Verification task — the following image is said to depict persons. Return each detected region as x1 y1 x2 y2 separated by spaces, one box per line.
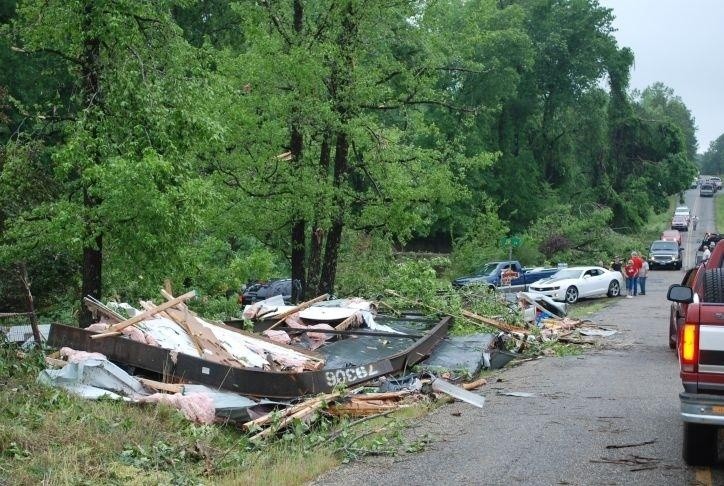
700 232 720 263
692 215 700 231
611 251 650 297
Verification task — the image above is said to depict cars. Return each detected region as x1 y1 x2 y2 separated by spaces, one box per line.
671 206 690 231
691 176 722 197
240 279 314 304
649 239 685 269
660 229 681 244
529 266 623 303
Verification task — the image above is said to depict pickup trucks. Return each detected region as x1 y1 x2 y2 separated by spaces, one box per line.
453 261 558 295
668 239 724 466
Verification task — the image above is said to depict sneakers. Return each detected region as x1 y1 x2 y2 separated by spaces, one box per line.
639 291 645 296
